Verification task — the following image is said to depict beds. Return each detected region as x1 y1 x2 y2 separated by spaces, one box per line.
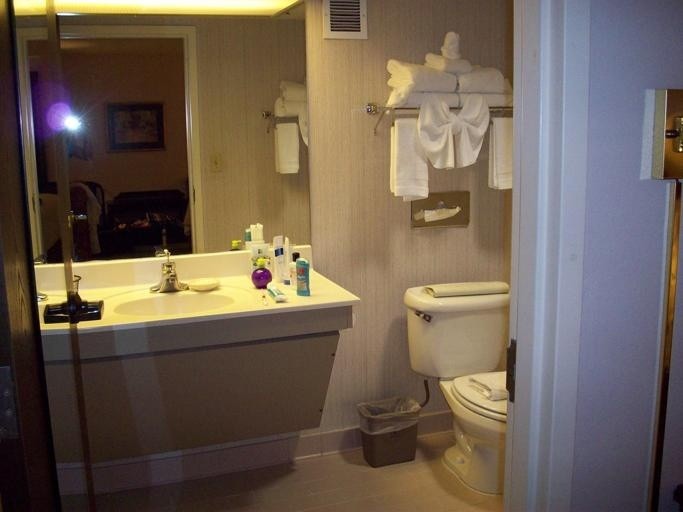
37 179 100 257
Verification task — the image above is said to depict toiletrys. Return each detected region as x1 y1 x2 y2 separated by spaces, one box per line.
228 222 266 249
249 235 311 306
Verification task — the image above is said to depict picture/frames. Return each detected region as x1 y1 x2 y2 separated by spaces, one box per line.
103 100 167 154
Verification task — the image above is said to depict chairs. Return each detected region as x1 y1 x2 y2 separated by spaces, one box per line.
83 181 106 221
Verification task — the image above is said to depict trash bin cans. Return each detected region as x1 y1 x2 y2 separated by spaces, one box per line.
356 395 421 467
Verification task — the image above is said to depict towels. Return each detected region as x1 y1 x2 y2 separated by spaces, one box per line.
466 371 506 401
270 75 310 177
381 25 514 205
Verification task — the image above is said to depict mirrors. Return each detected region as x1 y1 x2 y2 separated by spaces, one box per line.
15 23 205 264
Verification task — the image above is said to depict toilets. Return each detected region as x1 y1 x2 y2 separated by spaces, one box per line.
404 281 510 495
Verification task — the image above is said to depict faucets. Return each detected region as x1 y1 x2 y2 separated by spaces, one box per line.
153 245 170 256
162 272 178 286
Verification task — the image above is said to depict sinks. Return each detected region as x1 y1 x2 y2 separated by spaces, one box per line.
113 291 235 318
